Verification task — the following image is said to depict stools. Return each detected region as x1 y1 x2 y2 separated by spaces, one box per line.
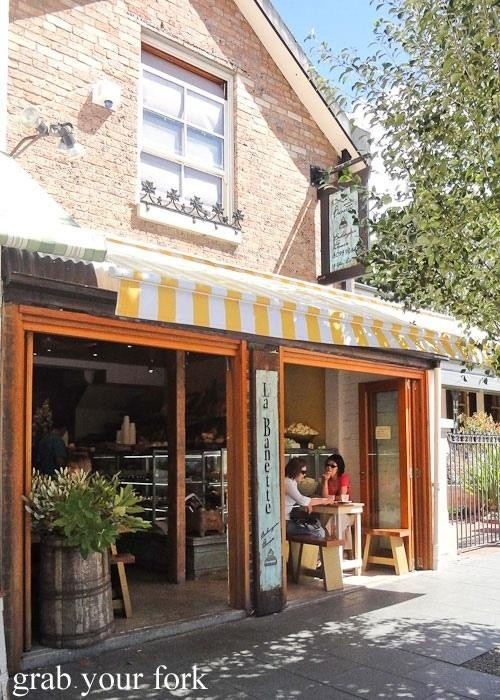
363 526 410 576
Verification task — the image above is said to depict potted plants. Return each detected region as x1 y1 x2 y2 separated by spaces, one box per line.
20 460 152 649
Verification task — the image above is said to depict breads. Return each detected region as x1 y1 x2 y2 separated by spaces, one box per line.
201 429 224 444
298 477 314 484
284 423 319 436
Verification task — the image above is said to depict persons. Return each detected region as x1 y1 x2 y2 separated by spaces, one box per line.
67 454 93 480
320 455 356 539
284 458 328 579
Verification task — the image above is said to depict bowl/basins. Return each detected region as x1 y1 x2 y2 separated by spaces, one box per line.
285 433 319 448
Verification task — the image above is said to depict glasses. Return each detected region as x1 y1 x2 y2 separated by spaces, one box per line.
325 463 338 468
301 470 308 475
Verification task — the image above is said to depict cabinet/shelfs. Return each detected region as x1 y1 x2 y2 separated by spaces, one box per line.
94 451 335 581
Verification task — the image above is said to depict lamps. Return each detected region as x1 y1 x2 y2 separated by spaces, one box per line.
10 106 85 162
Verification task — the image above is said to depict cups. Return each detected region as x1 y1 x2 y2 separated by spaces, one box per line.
328 495 336 501
341 495 349 502
115 415 137 445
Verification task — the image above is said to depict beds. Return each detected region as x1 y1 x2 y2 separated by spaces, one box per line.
300 502 365 578
